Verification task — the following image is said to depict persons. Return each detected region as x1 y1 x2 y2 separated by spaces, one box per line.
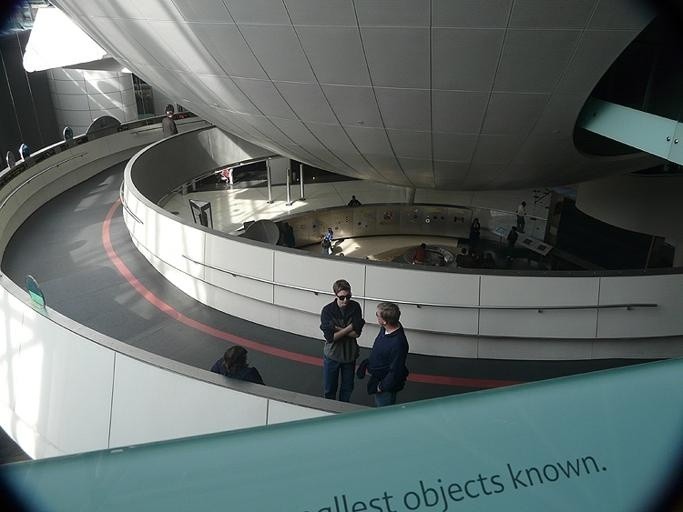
455 247 473 267
160 110 178 138
325 231 333 255
282 221 296 248
211 345 266 385
478 253 496 268
323 227 332 242
410 242 427 265
318 279 366 403
355 301 409 408
346 195 361 207
469 217 481 239
504 225 518 247
517 201 527 233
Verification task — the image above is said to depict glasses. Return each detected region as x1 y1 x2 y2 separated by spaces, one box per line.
336 294 351 300
